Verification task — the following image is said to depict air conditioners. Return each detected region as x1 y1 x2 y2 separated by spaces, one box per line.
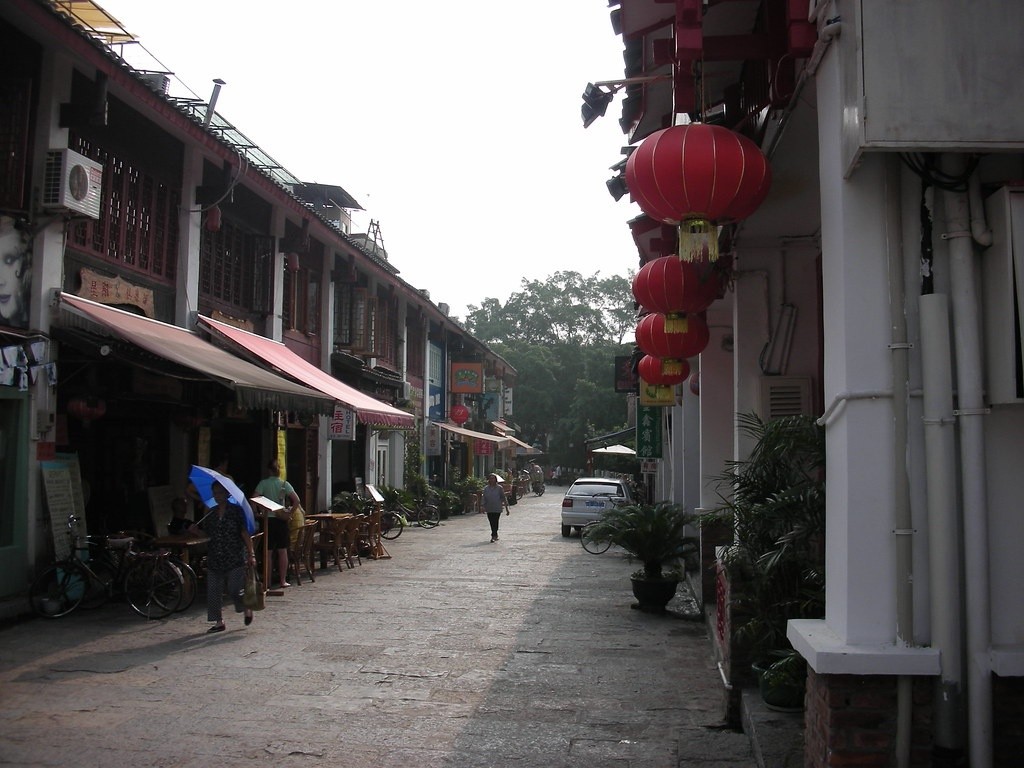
40 147 102 220
295 409 320 427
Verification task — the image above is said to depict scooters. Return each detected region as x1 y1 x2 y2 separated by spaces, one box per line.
515 471 546 498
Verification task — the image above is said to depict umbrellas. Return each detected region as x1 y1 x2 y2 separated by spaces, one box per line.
183 464 257 538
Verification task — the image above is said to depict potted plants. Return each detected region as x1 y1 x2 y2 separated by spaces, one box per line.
583 496 702 606
698 408 830 711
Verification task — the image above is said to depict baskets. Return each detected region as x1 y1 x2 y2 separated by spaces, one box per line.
48 533 74 567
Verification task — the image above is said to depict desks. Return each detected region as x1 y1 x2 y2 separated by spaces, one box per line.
306 512 351 568
151 535 210 602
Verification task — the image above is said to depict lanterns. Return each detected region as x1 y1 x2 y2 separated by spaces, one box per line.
637 355 699 399
631 254 720 332
207 205 221 232
288 251 298 272
450 405 468 424
625 122 772 262
635 314 710 376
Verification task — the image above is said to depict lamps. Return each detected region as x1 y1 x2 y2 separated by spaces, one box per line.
636 305 648 318
581 73 673 128
607 175 626 202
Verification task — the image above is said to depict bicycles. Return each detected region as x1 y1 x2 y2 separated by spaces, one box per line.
29 512 183 624
376 488 441 531
346 490 404 540
62 532 199 614
580 497 639 554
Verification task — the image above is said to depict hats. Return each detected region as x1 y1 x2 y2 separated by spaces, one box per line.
254 455 279 474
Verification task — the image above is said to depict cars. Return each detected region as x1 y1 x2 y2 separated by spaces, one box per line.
561 477 636 537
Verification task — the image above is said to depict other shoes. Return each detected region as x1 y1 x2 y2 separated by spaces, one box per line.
209 626 225 633
244 609 253 625
281 582 291 587
491 537 499 542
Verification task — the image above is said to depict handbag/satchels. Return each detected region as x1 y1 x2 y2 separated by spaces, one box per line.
235 570 264 613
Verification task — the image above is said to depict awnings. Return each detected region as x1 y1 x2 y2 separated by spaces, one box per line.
432 421 533 450
584 427 636 452
197 314 416 427
60 292 335 419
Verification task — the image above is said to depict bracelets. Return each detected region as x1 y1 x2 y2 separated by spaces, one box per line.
247 552 256 557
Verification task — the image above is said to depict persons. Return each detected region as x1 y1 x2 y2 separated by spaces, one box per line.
505 463 561 493
480 475 510 542
188 480 256 633
185 452 233 515
0 214 33 330
605 472 637 493
252 460 306 588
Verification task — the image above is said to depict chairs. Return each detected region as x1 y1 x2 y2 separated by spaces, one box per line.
250 510 386 586
